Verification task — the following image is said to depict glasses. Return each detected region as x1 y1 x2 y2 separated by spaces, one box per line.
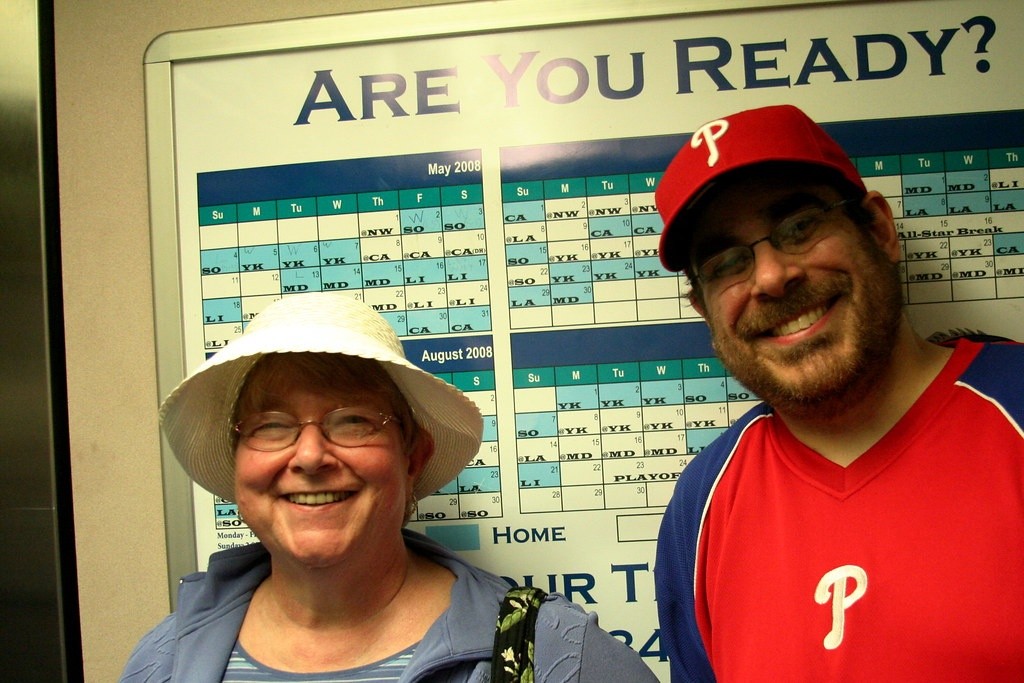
692 193 856 299
230 406 405 453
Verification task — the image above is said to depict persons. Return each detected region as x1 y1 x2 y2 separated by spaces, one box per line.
654 105 1024 683
118 292 660 683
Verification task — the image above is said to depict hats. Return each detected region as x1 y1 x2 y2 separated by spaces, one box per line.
153 289 485 504
652 104 870 272
